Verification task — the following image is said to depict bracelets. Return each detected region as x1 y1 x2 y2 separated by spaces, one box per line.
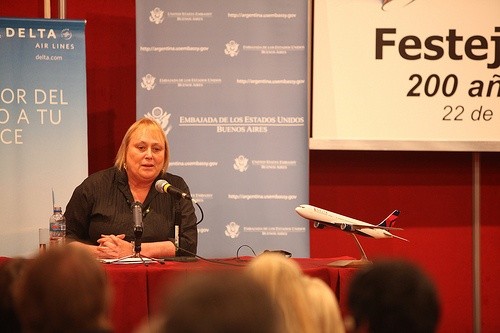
131 242 136 255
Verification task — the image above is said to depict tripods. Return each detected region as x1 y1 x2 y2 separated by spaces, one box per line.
107 221 164 267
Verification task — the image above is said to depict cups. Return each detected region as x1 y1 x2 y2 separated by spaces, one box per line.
38 228 49 252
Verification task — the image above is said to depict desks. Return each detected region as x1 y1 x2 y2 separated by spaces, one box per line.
0 256 360 333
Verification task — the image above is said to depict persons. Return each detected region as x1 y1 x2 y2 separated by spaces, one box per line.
249 254 346 333
346 259 440 333
0 247 104 333
60 118 198 259
167 267 286 333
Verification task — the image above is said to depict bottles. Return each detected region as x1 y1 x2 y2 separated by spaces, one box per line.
49 206 66 250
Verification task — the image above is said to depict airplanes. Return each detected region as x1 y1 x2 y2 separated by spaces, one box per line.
293 203 409 260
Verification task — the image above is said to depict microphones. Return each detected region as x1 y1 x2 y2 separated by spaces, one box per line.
130 201 145 236
155 179 192 200
